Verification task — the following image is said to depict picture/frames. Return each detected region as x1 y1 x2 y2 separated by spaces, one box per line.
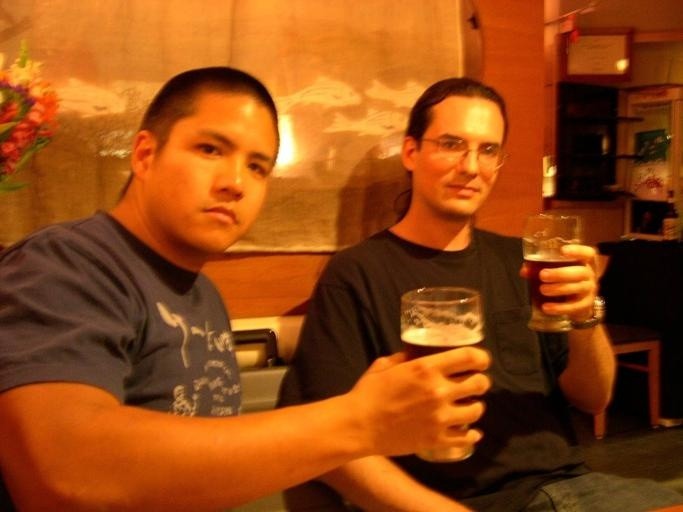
558 26 634 85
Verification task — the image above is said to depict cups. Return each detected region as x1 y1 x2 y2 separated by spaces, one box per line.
520 211 581 333
398 284 487 466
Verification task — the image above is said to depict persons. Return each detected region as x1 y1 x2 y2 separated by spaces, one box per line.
0 65 494 512
274 73 682 512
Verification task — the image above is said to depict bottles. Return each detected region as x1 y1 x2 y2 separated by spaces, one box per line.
661 189 681 241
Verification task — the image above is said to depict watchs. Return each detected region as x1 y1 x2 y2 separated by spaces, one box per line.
569 295 607 333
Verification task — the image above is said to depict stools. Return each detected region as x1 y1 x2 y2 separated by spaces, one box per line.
592 323 663 441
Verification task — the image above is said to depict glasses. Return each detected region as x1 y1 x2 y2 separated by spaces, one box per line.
416 135 508 172
